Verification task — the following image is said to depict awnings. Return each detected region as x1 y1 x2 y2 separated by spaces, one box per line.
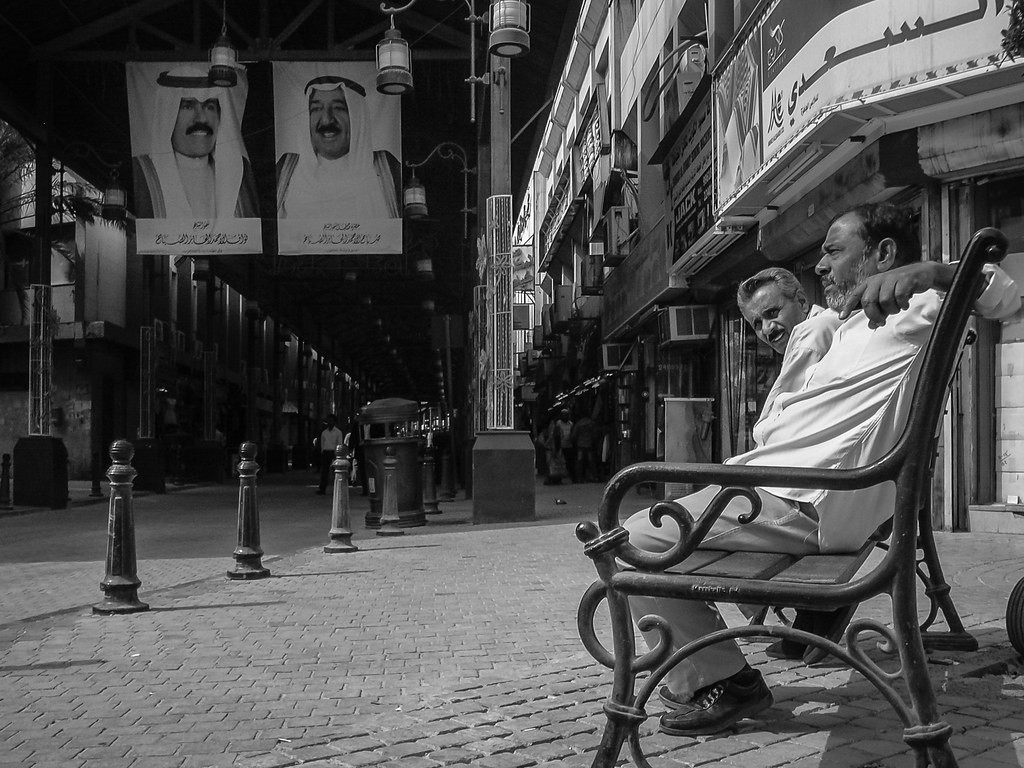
547 372 615 412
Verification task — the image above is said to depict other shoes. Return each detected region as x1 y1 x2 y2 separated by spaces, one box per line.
765 602 860 665
659 662 773 736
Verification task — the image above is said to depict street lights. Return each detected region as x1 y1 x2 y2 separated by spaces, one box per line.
374 0 535 525
405 141 489 498
12 140 127 508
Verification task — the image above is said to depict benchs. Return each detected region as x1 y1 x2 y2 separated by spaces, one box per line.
575 222 1009 768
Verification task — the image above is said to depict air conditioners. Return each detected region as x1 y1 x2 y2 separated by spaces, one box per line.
602 342 638 370
658 303 717 345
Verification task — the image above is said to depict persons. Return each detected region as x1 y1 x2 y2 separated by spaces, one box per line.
131 66 256 219
276 74 402 219
738 269 858 665
536 408 598 483
315 415 343 494
616 203 1022 735
344 413 371 496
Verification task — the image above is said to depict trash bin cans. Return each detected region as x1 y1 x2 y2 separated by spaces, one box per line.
421 456 443 515
359 397 427 530
437 455 458 503
